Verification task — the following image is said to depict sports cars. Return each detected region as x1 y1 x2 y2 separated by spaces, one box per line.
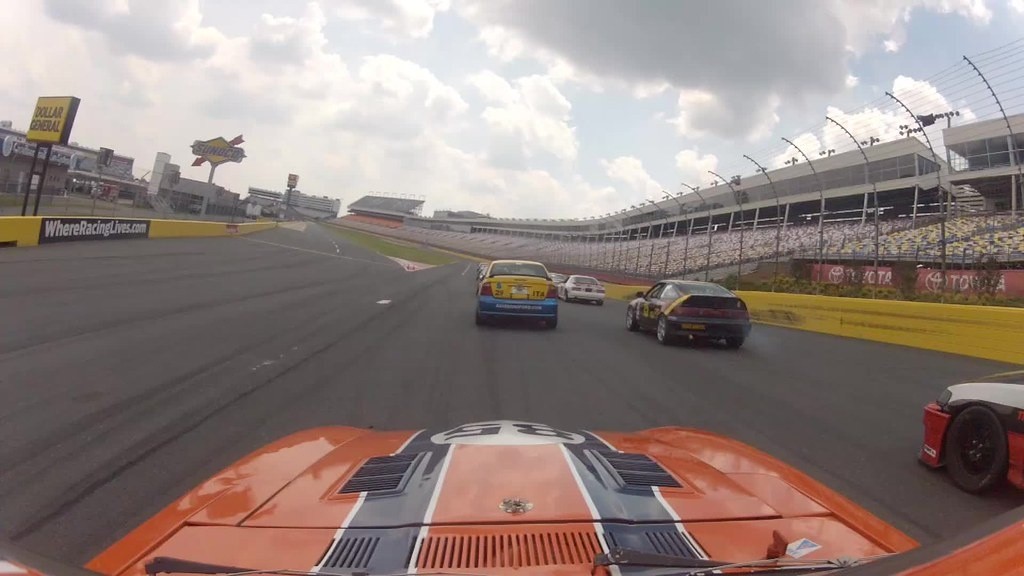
917 365 1024 498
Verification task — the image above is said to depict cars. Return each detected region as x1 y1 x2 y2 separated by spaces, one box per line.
626 280 753 346
474 262 490 292
549 272 567 291
557 274 607 306
474 260 560 330
